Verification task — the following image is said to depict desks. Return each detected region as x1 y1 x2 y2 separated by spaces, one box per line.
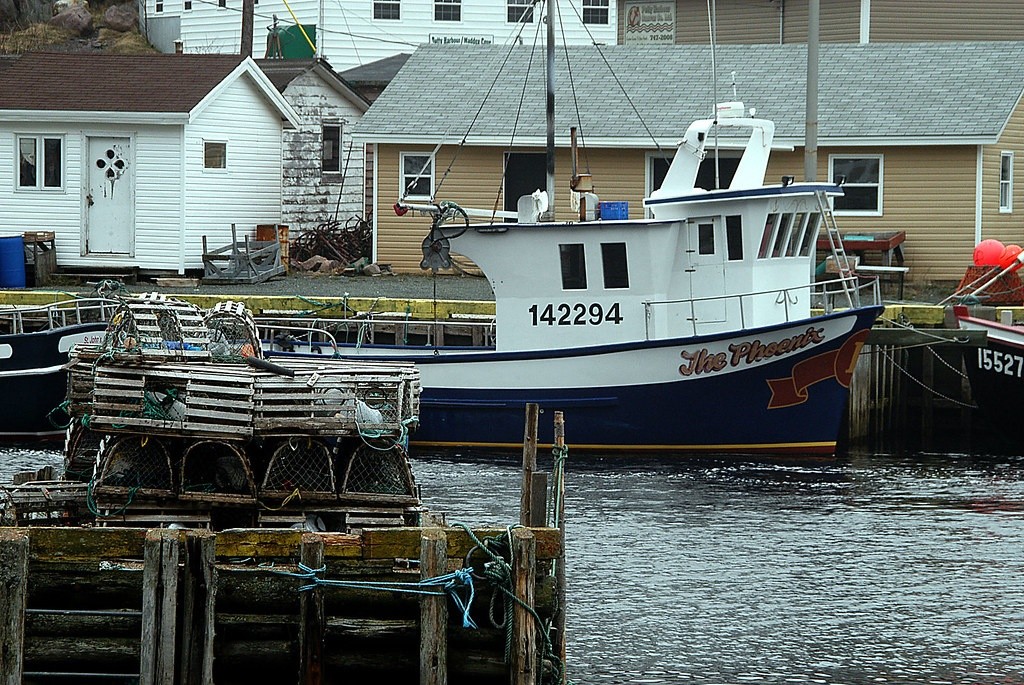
814 230 909 300
202 223 286 284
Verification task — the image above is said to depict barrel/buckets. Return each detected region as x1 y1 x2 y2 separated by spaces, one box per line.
0 236 26 288
257 225 289 277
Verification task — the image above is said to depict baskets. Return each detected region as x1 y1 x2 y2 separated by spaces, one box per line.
600 201 628 220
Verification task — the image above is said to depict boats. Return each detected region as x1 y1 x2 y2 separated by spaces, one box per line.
0 295 132 445
942 253 1024 460
129 1 888 469
